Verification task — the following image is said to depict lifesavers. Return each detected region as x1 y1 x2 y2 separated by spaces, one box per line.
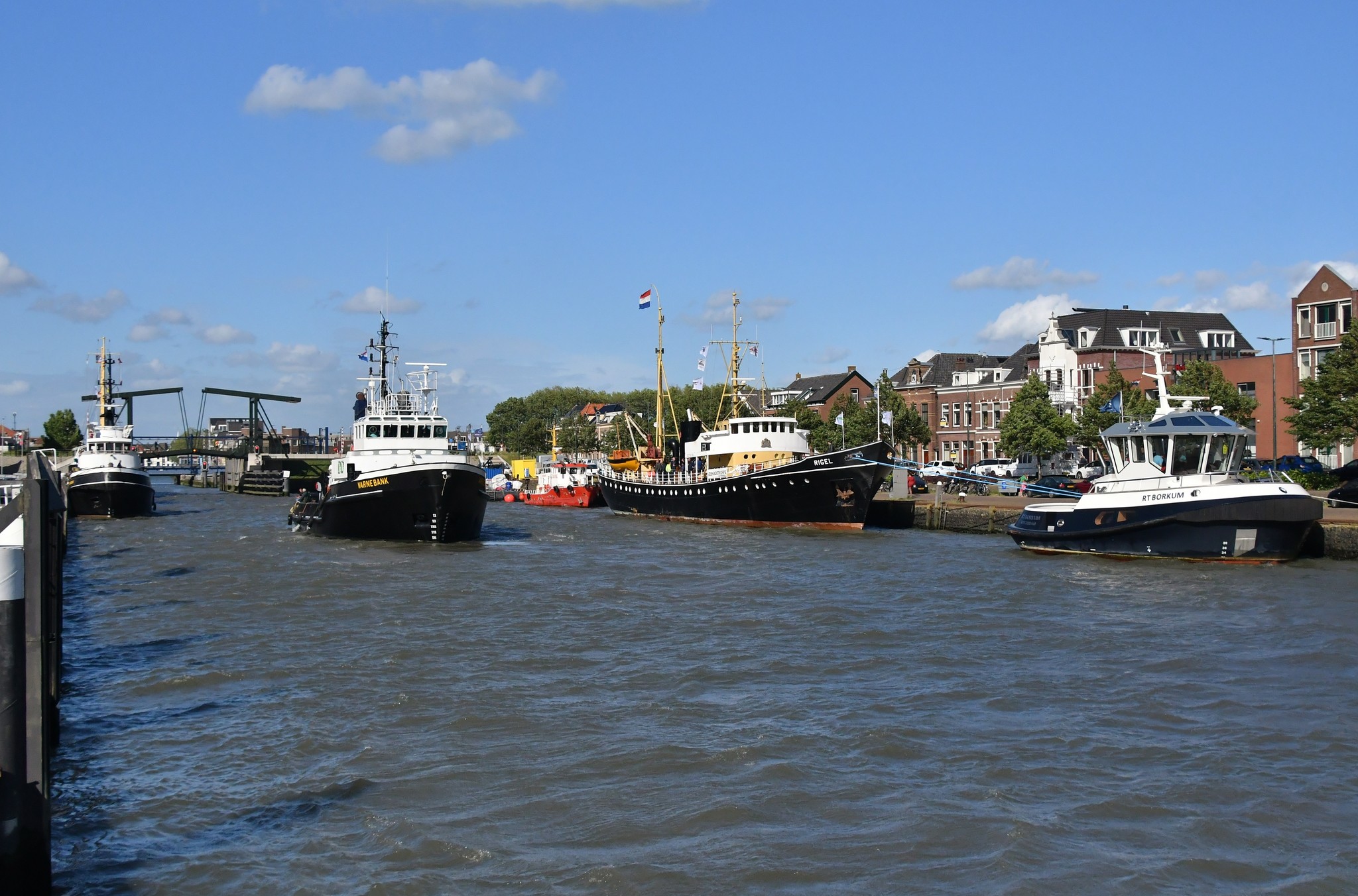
554 486 558 493
296 512 302 523
567 485 573 492
308 516 313 527
584 484 590 489
288 515 293 525
594 484 598 489
527 493 530 499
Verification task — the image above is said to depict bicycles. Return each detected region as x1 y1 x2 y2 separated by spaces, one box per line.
974 478 990 496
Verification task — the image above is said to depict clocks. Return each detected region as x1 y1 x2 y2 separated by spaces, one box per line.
1321 282 1328 292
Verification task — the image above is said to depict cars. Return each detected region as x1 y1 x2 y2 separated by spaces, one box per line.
970 458 1013 476
895 459 920 471
1073 474 1102 500
918 460 958 478
954 462 966 471
1074 459 1116 479
1229 454 1334 475
890 474 929 494
1024 476 1077 498
1328 458 1358 482
1327 478 1358 508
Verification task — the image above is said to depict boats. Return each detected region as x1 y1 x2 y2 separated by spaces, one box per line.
286 252 602 544
61 336 157 520
594 281 897 531
1005 320 1326 563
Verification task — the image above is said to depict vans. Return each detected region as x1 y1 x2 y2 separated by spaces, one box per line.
1005 445 1080 479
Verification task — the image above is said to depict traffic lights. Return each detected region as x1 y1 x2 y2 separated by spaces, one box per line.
254 445 260 455
202 461 208 470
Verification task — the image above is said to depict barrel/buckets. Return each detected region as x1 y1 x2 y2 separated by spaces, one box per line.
506 482 511 489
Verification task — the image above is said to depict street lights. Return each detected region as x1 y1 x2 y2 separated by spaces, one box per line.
1257 337 1291 470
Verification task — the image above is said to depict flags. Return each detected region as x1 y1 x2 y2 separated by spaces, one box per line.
835 412 844 426
639 289 652 309
1131 378 1141 387
749 345 759 358
873 388 879 399
358 350 368 362
692 376 704 391
880 411 893 428
474 428 483 439
697 358 706 372
699 345 709 358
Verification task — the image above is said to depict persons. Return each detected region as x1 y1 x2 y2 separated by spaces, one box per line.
889 477 893 492
165 442 168 452
907 473 915 497
154 441 158 452
941 473 959 494
117 460 122 468
295 488 310 504
1020 480 1028 497
1153 453 1166 468
215 439 219 450
828 440 835 453
654 457 707 485
1018 473 1028 496
1099 391 1123 415
